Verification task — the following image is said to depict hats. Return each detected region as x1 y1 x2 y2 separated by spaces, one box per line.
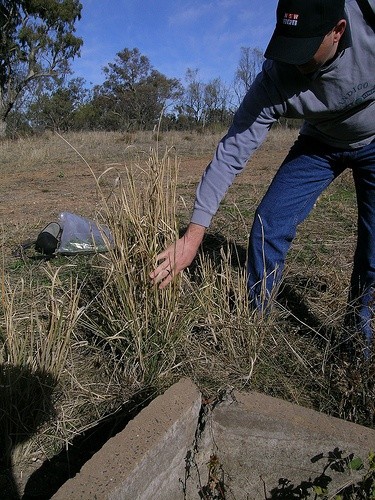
264 1 346 64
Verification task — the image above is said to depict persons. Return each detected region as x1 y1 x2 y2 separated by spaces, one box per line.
148 0 375 364
